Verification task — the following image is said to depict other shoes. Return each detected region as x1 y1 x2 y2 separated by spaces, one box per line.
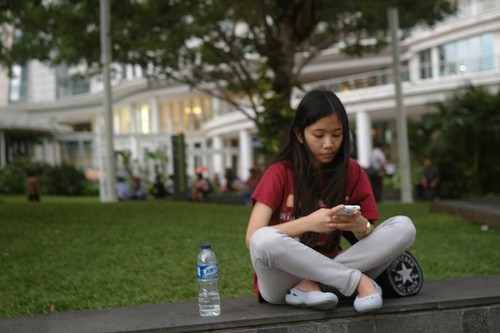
284 287 338 309
354 277 383 313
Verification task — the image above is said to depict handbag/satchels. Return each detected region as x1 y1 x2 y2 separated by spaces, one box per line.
376 250 424 298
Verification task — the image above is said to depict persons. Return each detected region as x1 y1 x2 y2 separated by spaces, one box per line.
115 142 440 203
246 90 417 311
27 171 40 200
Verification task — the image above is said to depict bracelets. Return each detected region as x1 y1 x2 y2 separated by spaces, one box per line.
357 220 371 236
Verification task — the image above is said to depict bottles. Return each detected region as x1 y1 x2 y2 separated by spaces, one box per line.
196 244 221 317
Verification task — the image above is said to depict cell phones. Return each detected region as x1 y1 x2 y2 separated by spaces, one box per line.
336 206 360 216
